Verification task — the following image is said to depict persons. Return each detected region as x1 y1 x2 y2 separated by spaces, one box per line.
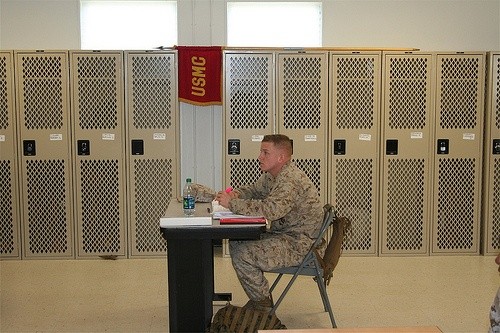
216 133 324 313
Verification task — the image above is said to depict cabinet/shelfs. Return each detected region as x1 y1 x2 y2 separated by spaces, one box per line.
223 45 500 260
0 48 179 257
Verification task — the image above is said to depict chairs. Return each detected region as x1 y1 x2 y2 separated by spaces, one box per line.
269 203 337 328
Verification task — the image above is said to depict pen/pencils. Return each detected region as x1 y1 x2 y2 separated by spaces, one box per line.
207 207 210 213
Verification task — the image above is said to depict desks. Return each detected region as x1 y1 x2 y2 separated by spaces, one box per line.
160 199 270 333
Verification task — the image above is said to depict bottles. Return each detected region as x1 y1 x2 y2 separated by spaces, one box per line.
182 178 196 216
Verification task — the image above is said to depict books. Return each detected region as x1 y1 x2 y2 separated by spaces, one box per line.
212 201 263 219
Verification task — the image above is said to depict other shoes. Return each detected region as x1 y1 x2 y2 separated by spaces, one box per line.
241 299 275 316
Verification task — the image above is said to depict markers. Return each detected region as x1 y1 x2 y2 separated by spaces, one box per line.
215 187 233 201
